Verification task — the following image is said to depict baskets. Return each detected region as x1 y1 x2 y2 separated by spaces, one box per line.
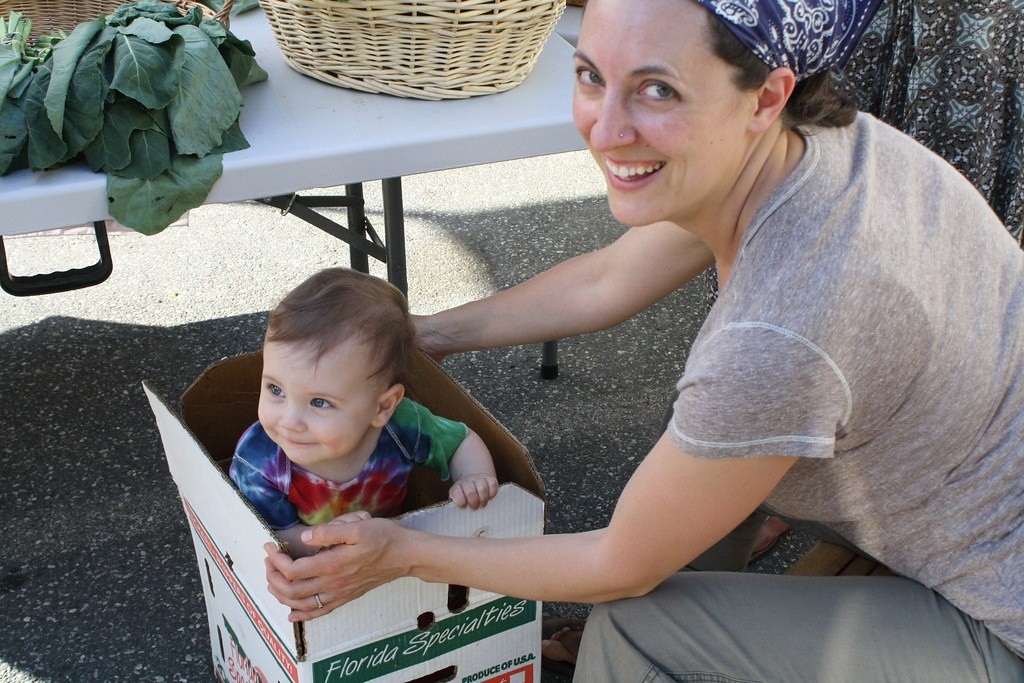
0 0 233 49
258 0 566 100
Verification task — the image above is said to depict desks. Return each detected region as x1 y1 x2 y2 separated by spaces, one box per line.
0 6 590 315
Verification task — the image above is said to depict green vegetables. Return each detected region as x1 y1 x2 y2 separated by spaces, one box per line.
0 0 268 238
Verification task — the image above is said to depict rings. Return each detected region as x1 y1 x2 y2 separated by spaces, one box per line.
314 594 323 608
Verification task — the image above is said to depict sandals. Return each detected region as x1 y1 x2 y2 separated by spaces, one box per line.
542 618 586 675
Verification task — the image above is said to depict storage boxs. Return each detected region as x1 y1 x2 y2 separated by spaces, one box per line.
141 348 548 683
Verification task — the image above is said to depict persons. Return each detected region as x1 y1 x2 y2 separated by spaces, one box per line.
263 0 1024 682
228 267 499 558
750 0 1024 574
